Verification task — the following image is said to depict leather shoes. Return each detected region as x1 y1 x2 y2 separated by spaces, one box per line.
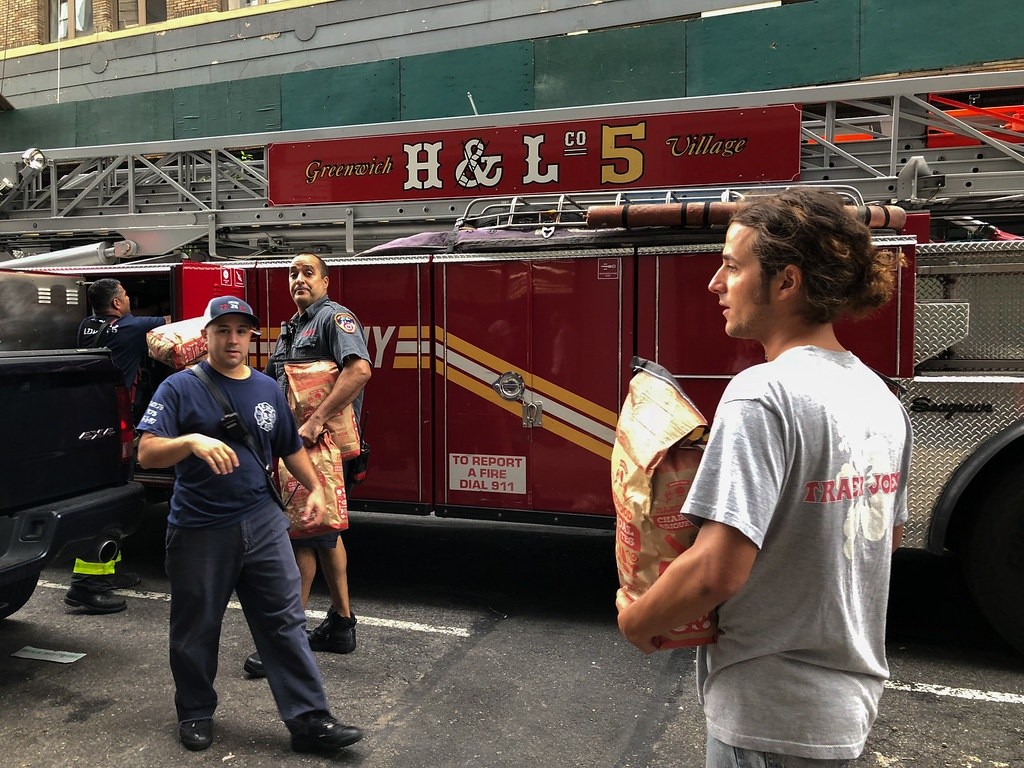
179 719 215 752
286 709 365 752
244 651 266 677
306 605 357 653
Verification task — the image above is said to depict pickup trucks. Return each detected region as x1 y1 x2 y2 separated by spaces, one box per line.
0 346 146 623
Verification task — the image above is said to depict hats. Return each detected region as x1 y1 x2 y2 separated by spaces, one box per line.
203 295 261 329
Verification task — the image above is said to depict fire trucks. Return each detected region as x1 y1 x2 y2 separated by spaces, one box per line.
0 69 1022 668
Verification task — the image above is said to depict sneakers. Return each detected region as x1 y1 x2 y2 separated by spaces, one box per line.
64 573 127 612
101 563 141 589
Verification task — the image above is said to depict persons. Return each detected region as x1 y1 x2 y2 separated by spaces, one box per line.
135 294 364 756
241 249 375 676
615 186 916 768
63 279 177 614
